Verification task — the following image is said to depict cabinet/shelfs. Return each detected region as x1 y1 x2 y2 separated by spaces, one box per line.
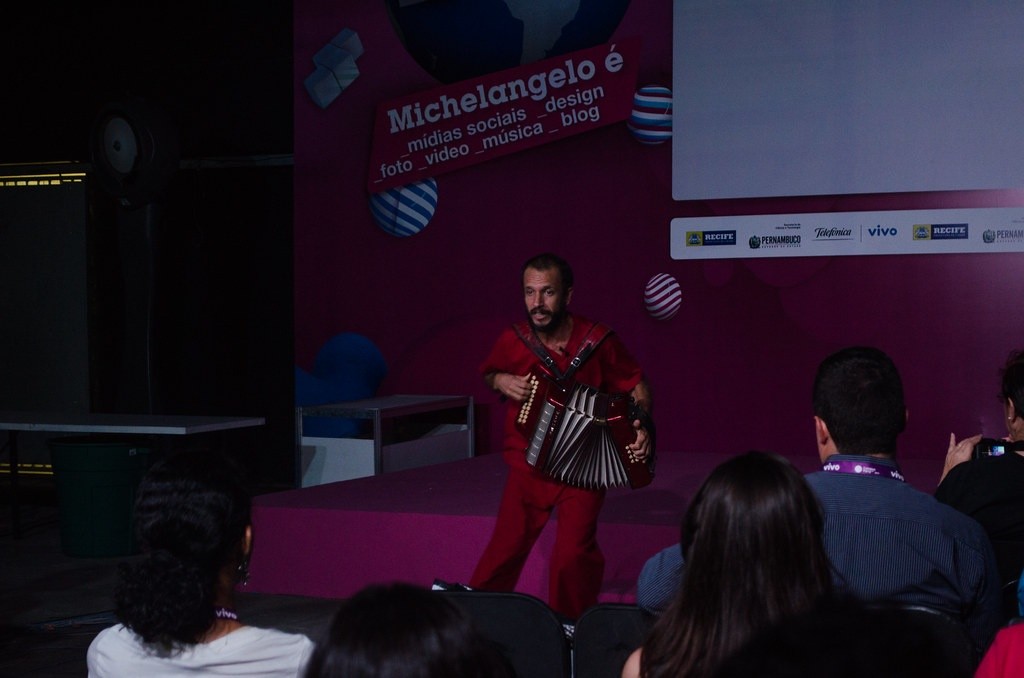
293 391 474 490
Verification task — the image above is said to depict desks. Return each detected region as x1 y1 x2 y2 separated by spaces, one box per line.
0 408 265 541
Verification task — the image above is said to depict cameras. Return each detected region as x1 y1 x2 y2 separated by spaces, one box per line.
972 438 1013 461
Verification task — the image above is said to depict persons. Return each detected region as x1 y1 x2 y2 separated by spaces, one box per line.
622 449 830 678
636 347 1024 678
86 450 317 678
478 253 654 642
934 351 1024 619
301 583 516 678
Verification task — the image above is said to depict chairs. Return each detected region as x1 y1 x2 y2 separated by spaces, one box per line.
573 604 653 678
433 588 571 678
863 604 977 678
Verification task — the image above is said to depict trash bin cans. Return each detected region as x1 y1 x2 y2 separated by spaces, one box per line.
45 437 140 556
